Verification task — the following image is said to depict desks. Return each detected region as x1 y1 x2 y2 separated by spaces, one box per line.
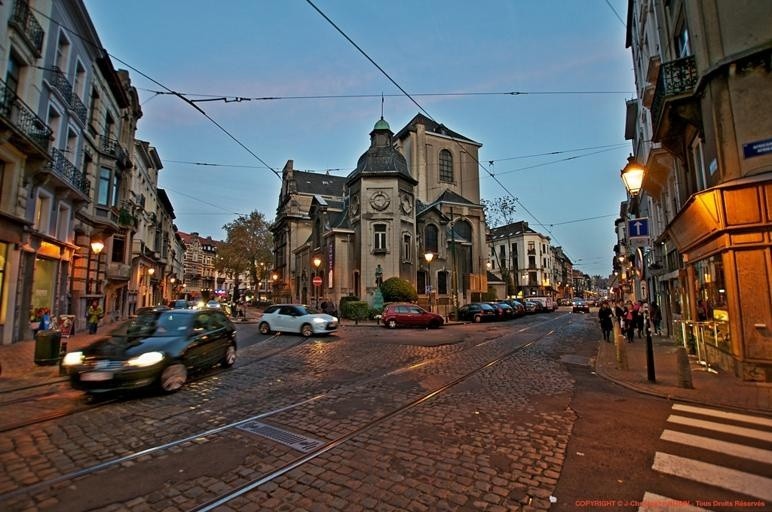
673 320 718 375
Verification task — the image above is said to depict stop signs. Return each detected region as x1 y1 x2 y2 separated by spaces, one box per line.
312 276 323 286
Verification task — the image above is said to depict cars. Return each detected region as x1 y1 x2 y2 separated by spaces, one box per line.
257 303 338 336
59 299 237 395
573 301 589 312
383 303 444 328
446 296 558 322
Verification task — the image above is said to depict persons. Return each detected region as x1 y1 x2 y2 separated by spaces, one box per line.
698 299 713 320
87 300 103 333
598 299 662 344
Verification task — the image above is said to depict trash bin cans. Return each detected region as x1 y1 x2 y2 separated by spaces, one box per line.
33 329 61 366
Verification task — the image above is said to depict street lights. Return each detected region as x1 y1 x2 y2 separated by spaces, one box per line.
313 259 321 308
620 153 655 381
424 250 434 311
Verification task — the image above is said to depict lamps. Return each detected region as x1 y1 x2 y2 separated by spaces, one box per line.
91 240 104 254
